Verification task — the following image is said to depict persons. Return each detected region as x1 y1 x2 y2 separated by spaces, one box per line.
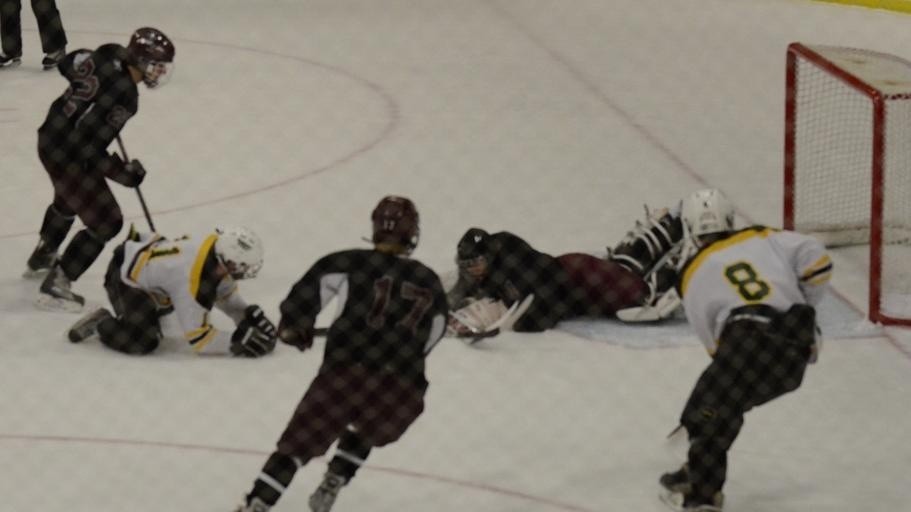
234 192 449 512
657 187 835 512
0 0 68 69
26 26 176 307
435 197 700 344
68 224 277 356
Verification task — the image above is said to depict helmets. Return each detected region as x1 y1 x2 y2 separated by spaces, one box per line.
456 227 499 271
125 27 176 65
679 187 737 237
371 194 420 246
214 221 266 282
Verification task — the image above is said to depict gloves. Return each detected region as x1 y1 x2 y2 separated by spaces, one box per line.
276 301 315 353
105 152 148 187
230 305 277 359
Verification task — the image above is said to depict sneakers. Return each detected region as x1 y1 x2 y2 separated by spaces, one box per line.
27 237 71 271
42 46 65 67
0 47 23 64
309 475 341 510
660 462 724 510
68 308 112 343
39 262 84 307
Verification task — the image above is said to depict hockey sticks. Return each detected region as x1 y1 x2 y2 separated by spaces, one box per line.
313 326 498 340
